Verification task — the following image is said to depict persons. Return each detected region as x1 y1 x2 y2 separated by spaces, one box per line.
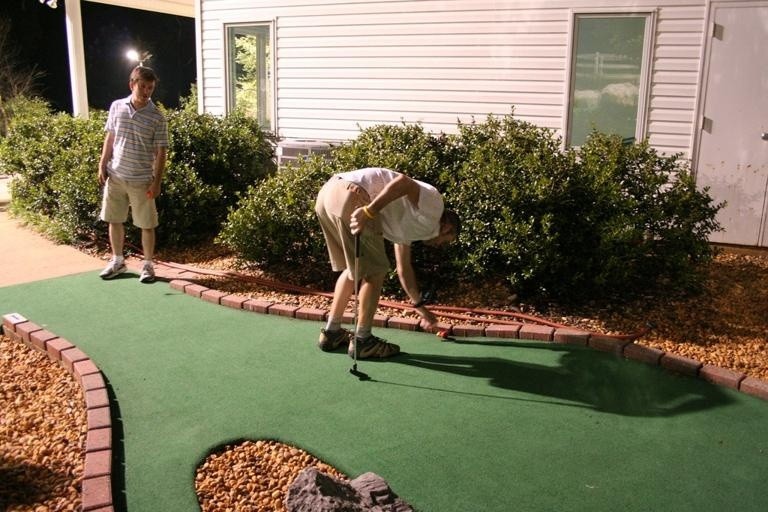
99 65 169 283
315 167 462 360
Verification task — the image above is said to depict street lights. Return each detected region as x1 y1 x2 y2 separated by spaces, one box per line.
126 50 142 67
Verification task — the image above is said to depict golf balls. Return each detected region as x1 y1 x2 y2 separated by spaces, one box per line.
441 331 448 338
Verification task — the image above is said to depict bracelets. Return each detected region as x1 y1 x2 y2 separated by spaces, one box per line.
408 297 424 308
363 205 376 220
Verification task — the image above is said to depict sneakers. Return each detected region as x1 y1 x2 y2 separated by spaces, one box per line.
318 326 355 352
347 334 400 360
99 262 126 279
139 265 155 283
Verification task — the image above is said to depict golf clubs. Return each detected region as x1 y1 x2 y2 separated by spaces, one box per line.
349 232 368 380
